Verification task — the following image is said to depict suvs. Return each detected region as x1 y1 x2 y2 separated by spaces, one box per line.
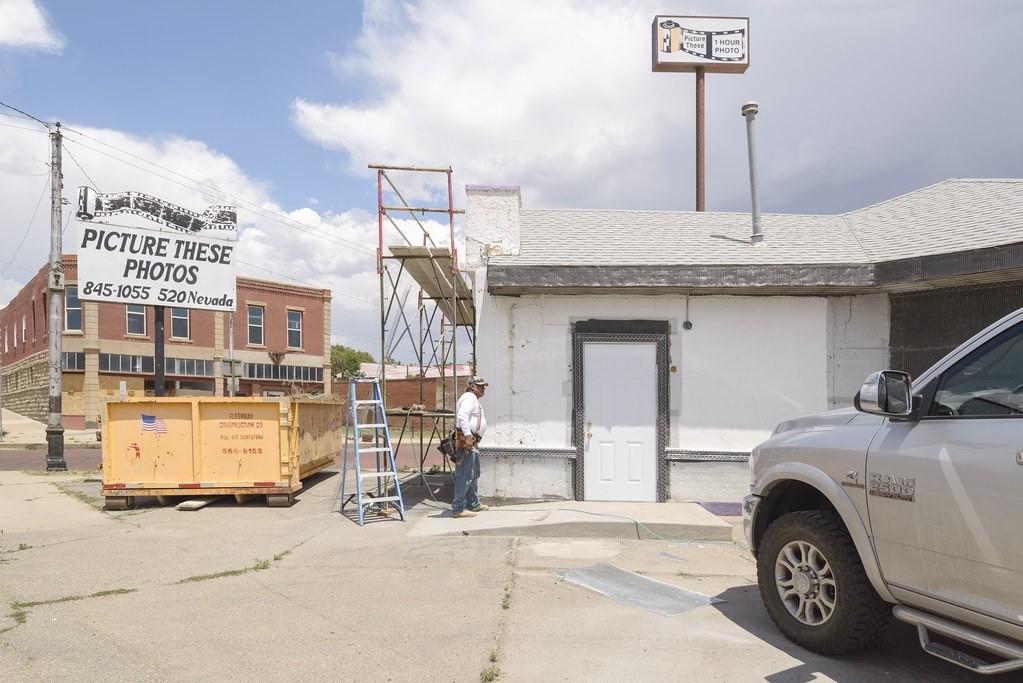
741 307 1023 676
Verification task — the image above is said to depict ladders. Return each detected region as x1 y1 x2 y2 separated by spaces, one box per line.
339 376 407 527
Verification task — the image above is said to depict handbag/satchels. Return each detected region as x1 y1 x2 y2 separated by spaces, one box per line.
438 430 456 464
455 431 474 449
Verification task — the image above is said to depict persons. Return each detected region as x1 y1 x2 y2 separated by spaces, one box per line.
452 376 489 517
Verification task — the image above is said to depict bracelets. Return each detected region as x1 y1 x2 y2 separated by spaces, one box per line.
465 436 471 439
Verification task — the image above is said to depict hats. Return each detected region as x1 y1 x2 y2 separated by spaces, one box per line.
468 376 488 386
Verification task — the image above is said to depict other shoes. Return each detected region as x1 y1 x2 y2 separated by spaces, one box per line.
454 511 476 517
469 503 489 511
